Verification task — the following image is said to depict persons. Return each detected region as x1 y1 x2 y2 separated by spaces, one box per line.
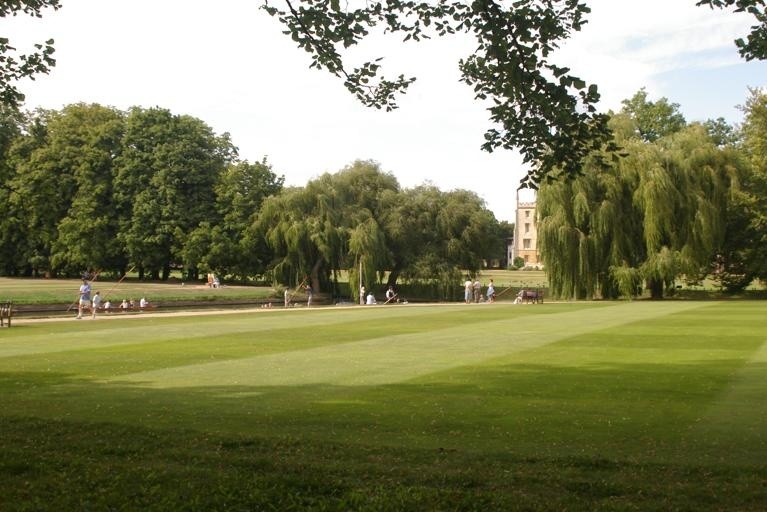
103 299 111 310
119 298 131 308
301 283 313 308
384 285 396 304
514 287 526 305
74 277 95 321
92 291 101 308
365 292 377 305
207 270 215 288
130 298 135 306
139 297 148 308
464 279 496 304
284 286 292 307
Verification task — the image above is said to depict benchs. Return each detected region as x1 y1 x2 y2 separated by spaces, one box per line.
523 290 544 304
0 302 13 327
